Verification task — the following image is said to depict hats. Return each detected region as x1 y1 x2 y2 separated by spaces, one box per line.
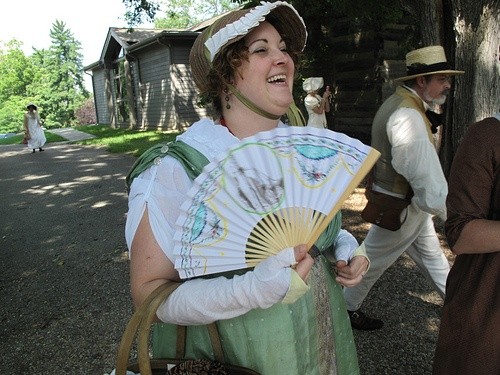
303 77 324 91
189 2 308 94
27 104 37 110
394 46 465 83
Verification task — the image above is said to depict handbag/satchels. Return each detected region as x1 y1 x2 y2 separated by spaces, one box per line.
360 189 410 232
23 132 30 144
106 281 260 375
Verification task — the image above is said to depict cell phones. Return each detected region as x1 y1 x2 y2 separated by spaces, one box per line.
326 86 329 92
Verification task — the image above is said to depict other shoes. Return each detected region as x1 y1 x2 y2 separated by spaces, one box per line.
39 149 45 152
32 149 35 154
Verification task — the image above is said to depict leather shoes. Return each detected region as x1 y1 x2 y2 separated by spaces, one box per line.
347 308 385 330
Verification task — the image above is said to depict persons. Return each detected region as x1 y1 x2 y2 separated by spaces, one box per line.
342 44 451 330
124 0 371 375
24 105 47 153
434 117 500 375
